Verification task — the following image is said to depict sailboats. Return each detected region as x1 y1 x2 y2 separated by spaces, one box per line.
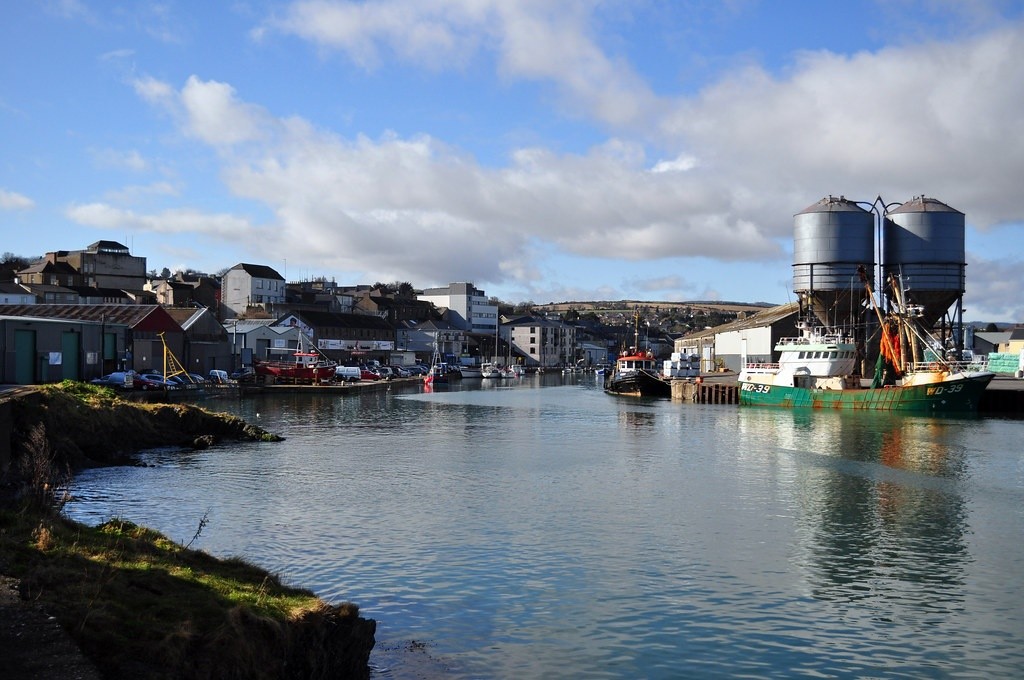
255 310 337 387
603 310 672 399
461 305 525 378
425 333 463 383
737 264 997 417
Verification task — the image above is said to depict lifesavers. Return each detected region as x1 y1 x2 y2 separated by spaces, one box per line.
929 362 938 370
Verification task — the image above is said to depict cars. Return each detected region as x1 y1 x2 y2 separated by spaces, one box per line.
336 361 431 382
90 370 239 390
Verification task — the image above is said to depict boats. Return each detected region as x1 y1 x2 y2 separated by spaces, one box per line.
561 367 611 375
